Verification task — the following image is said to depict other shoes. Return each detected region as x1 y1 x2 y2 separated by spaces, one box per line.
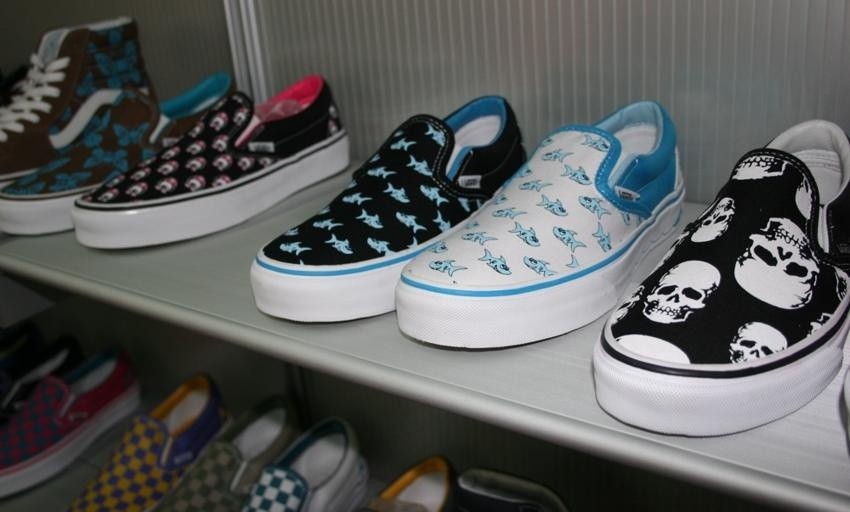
358 454 455 512
445 466 571 512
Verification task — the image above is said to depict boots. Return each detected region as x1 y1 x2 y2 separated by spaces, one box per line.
0 17 161 178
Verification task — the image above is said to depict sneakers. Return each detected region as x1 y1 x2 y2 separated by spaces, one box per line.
63 370 233 512
242 416 369 512
0 76 236 236
591 119 850 439
250 95 529 323
0 340 140 499
0 319 88 425
146 395 307 512
72 75 350 249
396 101 687 347
